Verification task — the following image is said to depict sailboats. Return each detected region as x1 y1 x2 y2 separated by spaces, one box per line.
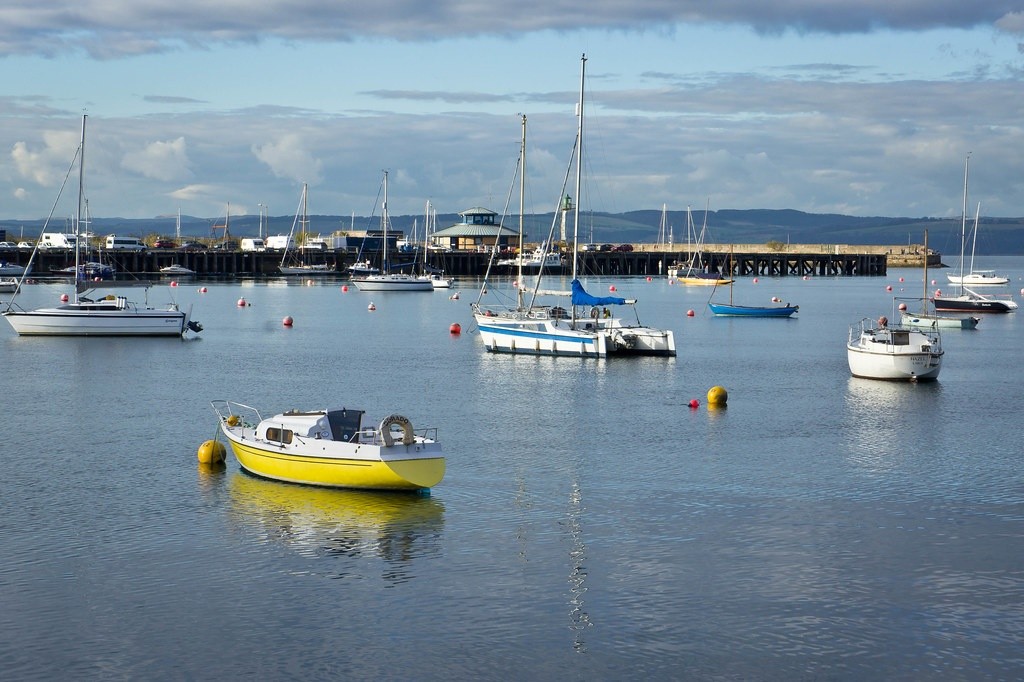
946 201 1011 285
469 53 677 360
668 198 736 285
277 182 331 275
0 115 204 337
929 151 1018 313
345 169 457 293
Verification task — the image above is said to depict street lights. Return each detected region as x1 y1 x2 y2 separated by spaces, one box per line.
258 204 268 239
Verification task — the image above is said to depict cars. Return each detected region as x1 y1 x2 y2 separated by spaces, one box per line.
596 243 614 252
611 243 633 252
0 241 19 253
18 242 40 254
213 240 239 251
154 239 178 248
182 239 207 251
582 243 597 251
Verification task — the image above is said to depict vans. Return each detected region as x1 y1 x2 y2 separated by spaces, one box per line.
105 236 147 252
41 232 80 254
263 235 296 253
241 238 265 252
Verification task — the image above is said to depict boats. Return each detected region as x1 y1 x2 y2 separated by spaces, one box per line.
0 262 33 276
160 264 197 276
209 399 447 492
0 277 21 294
896 229 981 330
49 261 116 280
846 296 945 381
707 243 800 318
